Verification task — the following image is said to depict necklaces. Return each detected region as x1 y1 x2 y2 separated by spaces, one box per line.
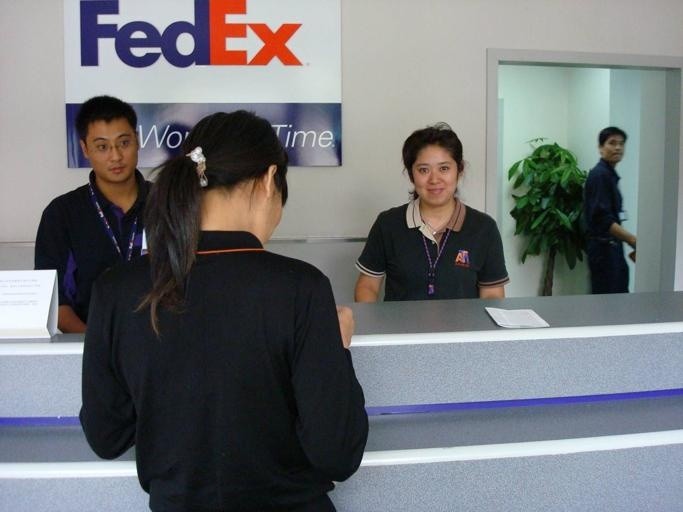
419 204 450 236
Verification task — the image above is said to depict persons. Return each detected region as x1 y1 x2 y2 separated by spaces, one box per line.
354 122 510 301
78 108 370 511
33 95 158 335
584 126 636 293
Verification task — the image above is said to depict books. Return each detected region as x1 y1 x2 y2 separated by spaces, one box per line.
485 304 551 329
